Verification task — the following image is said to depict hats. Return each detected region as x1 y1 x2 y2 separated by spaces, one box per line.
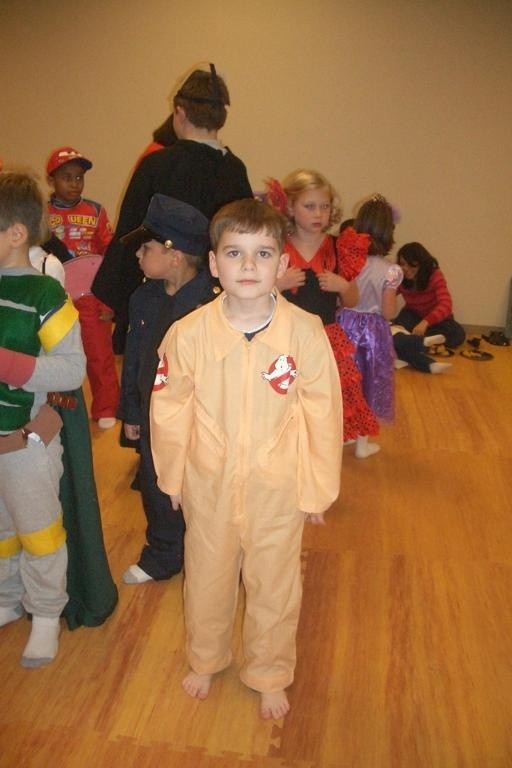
47 148 92 173
120 193 209 256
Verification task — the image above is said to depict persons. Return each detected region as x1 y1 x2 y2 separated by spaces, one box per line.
269 168 381 444
113 192 221 586
28 243 120 633
40 145 124 433
390 240 469 375
1 167 90 673
147 196 349 721
28 190 67 288
333 197 405 461
90 65 250 357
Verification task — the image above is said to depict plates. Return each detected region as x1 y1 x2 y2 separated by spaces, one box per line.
459 349 494 362
424 345 456 359
